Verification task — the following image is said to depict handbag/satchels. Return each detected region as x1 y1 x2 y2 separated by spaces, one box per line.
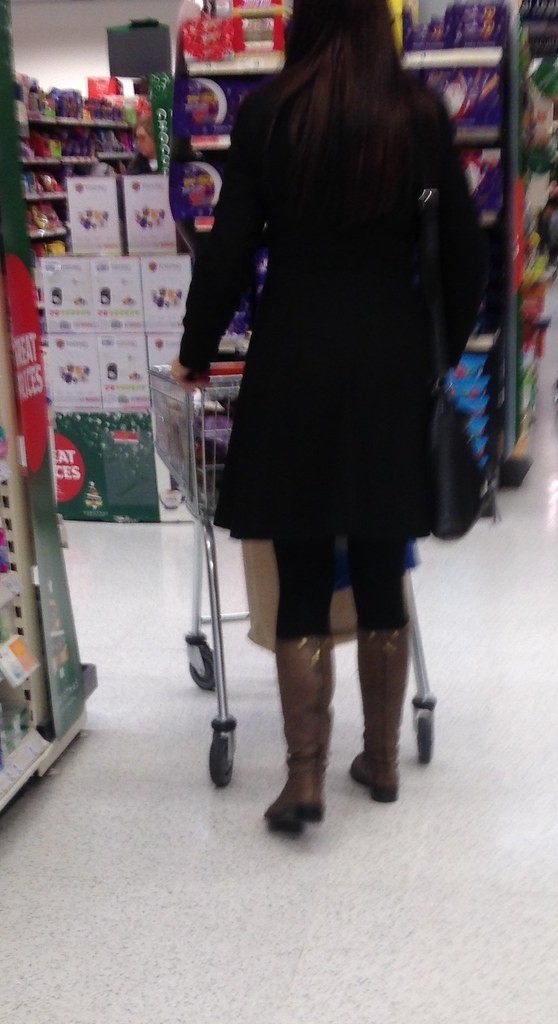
429 373 482 541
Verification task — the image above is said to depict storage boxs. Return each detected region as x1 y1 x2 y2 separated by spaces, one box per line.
15 2 508 412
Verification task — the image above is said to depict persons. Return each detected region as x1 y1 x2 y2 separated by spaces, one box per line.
171 1 486 824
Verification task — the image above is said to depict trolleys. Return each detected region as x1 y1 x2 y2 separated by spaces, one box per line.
150 348 438 788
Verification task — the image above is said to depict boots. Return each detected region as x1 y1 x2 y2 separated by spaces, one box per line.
352 623 409 802
264 636 334 837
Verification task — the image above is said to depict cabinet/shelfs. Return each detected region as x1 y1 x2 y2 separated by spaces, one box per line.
19 112 134 243
167 1 521 523
